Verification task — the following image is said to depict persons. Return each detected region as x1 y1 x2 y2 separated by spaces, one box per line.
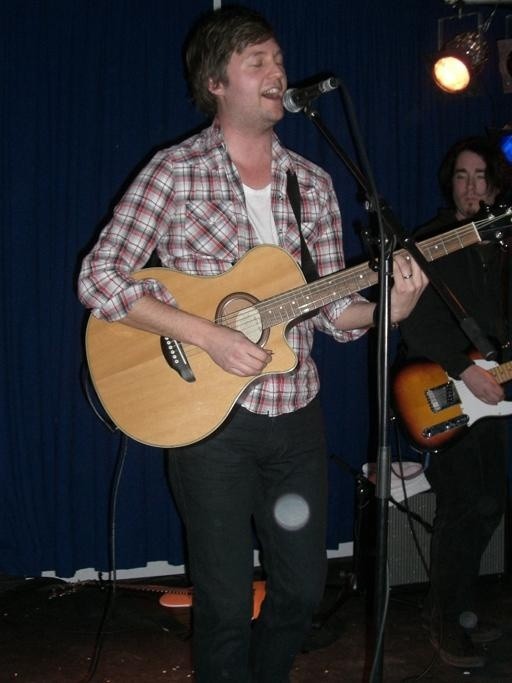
77 7 429 683
396 139 512 667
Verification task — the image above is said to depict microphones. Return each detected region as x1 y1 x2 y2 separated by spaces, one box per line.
281 75 337 114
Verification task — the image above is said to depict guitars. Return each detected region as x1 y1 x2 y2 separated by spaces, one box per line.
48 580 266 624
393 350 512 446
86 202 510 448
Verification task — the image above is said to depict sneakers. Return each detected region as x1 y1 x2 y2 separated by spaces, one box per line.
419 609 503 669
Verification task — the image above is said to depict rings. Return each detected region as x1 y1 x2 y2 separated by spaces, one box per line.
403 274 412 279
